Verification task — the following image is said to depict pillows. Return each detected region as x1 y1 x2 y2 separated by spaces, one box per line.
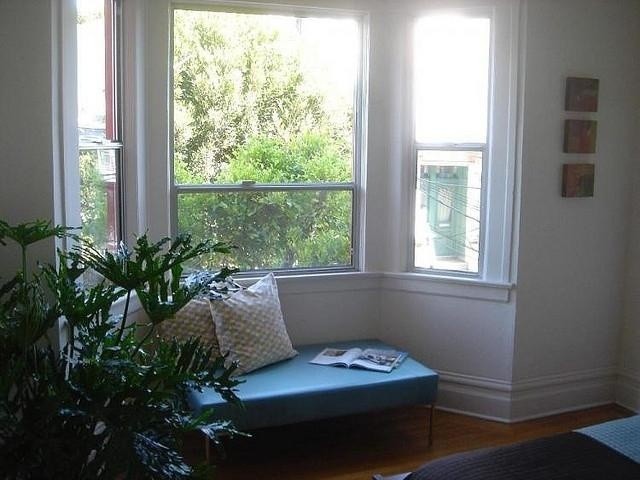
154 295 221 372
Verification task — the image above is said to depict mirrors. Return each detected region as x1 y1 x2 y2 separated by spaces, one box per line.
209 271 300 377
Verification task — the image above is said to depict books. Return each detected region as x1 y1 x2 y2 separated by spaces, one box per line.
309 347 402 373
387 350 409 368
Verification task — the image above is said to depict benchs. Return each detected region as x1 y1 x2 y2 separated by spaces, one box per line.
183 339 439 479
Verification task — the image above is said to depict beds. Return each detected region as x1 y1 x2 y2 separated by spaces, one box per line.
403 412 640 480
